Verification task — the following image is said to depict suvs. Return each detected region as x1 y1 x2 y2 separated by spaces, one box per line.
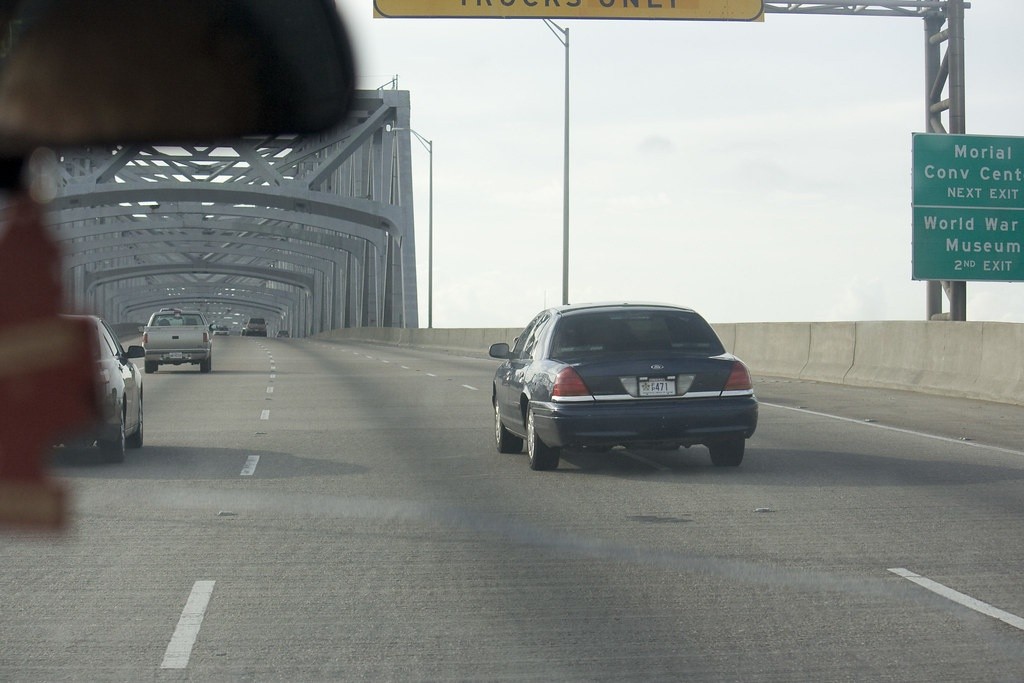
244 317 269 336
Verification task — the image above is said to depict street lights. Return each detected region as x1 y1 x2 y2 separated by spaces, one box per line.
392 127 433 330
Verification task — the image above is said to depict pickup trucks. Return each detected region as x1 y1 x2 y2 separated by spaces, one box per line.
137 307 217 373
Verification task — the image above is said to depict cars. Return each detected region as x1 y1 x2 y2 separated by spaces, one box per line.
487 302 759 470
212 325 232 336
275 330 290 338
0 315 147 465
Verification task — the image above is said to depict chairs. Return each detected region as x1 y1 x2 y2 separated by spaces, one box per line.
158 319 170 326
182 317 198 325
